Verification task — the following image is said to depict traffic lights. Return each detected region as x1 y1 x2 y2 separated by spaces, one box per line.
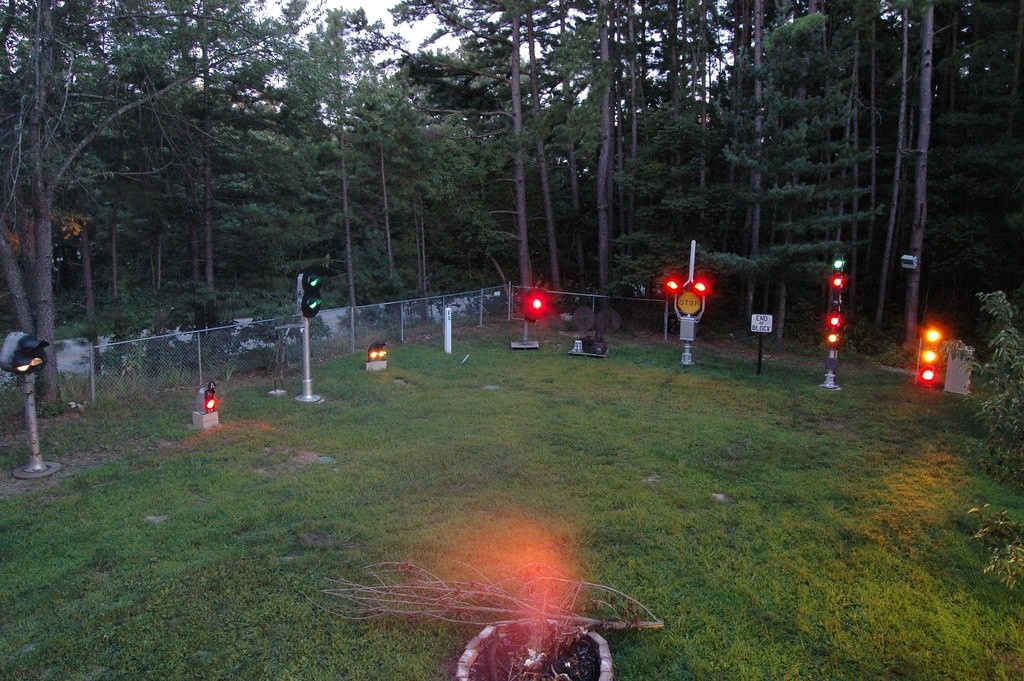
301 264 326 318
663 280 683 293
692 280 709 295
11 335 49 374
827 313 842 349
916 324 943 387
830 256 844 293
519 289 546 323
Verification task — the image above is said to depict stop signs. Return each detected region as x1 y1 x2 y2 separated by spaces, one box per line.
677 293 701 317
